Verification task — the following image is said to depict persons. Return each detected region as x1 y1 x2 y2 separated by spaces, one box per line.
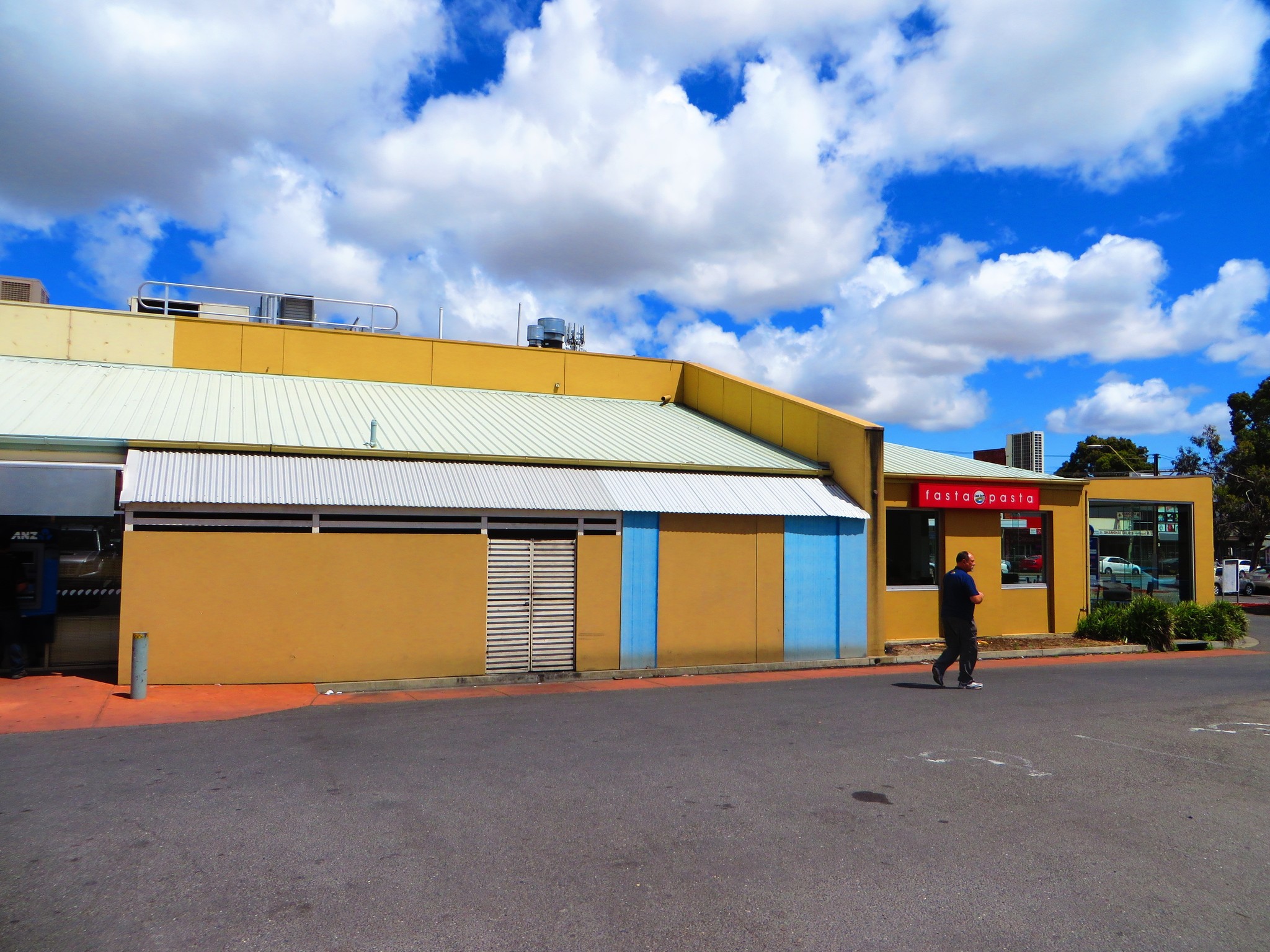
931 550 985 689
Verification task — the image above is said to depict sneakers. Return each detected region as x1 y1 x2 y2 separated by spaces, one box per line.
932 666 943 686
958 681 983 690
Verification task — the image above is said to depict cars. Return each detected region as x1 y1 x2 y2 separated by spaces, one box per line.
1214 558 1270 597
1001 559 1011 574
1099 556 1141 575
1018 555 1043 573
1158 558 1179 575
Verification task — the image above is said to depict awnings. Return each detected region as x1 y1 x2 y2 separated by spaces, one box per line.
0 460 123 517
119 446 874 524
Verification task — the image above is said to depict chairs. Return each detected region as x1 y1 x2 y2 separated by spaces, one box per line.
1103 583 1132 603
